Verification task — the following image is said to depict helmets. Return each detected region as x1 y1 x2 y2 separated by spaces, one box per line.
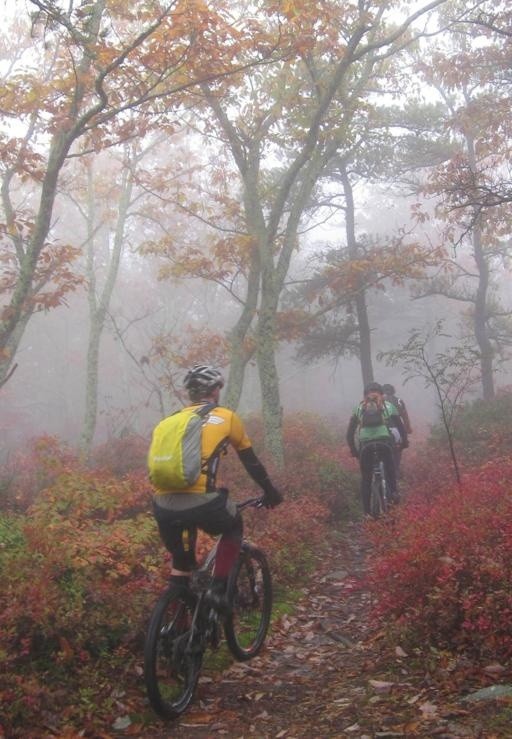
183 365 224 389
364 383 395 395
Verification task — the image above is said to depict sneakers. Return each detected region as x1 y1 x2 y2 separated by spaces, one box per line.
211 578 253 604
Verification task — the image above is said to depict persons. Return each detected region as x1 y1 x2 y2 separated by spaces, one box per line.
382 385 412 466
347 383 409 521
151 365 282 636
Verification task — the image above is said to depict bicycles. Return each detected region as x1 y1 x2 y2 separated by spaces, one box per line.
143 495 273 721
354 442 407 519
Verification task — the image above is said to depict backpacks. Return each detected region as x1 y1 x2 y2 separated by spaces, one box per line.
148 404 219 490
360 391 385 428
